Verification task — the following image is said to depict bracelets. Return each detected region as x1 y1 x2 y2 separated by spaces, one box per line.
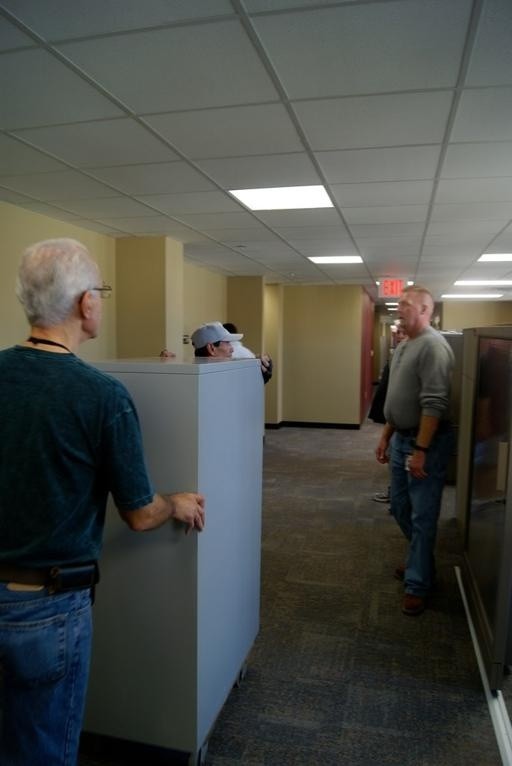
414 444 428 454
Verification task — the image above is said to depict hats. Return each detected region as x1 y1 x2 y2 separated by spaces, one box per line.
191 321 243 349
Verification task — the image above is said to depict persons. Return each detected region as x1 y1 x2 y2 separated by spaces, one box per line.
369 322 410 503
0 235 208 765
371 288 458 618
221 322 258 361
191 321 274 386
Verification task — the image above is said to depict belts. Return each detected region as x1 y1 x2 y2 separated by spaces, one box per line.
0 567 44 585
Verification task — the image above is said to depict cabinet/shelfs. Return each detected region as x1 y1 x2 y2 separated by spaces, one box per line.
89 358 267 765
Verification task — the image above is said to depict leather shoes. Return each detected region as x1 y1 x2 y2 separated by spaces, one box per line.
401 584 425 617
394 563 409 580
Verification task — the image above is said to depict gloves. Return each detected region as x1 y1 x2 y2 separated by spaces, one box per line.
261 356 272 384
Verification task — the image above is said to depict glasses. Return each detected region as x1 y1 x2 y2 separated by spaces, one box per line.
93 284 112 299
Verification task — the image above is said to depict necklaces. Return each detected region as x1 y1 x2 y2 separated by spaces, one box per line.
25 336 71 354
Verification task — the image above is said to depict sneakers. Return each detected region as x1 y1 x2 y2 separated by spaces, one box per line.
374 486 391 503
